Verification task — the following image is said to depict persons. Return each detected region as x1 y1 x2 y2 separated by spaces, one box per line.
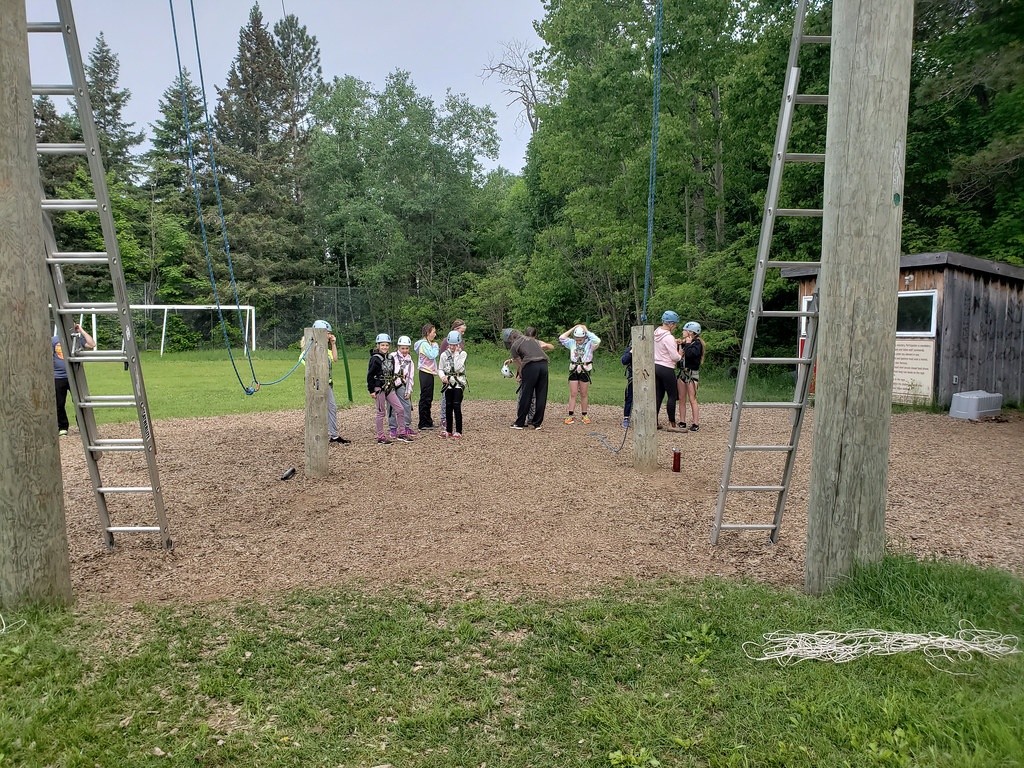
298 320 351 445
677 322 706 431
621 346 633 427
504 328 554 425
438 331 468 440
654 310 688 433
414 323 439 429
52 319 96 435
367 334 414 445
503 328 549 430
387 336 418 439
440 318 466 429
559 324 601 424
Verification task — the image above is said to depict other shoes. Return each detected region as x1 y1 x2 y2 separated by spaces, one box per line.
420 427 434 429
59 430 68 436
622 418 629 429
657 425 663 429
440 420 447 429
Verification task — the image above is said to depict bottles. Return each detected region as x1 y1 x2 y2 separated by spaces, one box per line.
281 468 295 480
673 448 681 472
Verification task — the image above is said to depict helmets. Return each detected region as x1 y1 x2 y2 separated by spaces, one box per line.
503 328 514 344
375 333 391 343
640 314 647 320
397 336 411 346
661 311 680 322
573 327 586 337
683 322 701 334
447 331 462 344
312 320 332 332
500 366 513 378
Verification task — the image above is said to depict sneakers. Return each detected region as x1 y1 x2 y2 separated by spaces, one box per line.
565 416 574 425
404 428 417 436
329 437 351 444
689 424 699 432
535 425 541 430
452 432 462 439
378 439 394 445
679 422 686 428
397 436 414 443
510 422 523 429
437 431 453 438
581 414 591 424
390 428 397 438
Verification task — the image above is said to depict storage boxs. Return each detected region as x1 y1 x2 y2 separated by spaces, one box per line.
948 390 1003 420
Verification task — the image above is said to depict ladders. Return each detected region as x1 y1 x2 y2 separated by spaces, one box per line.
711 1 831 546
24 1 173 549
